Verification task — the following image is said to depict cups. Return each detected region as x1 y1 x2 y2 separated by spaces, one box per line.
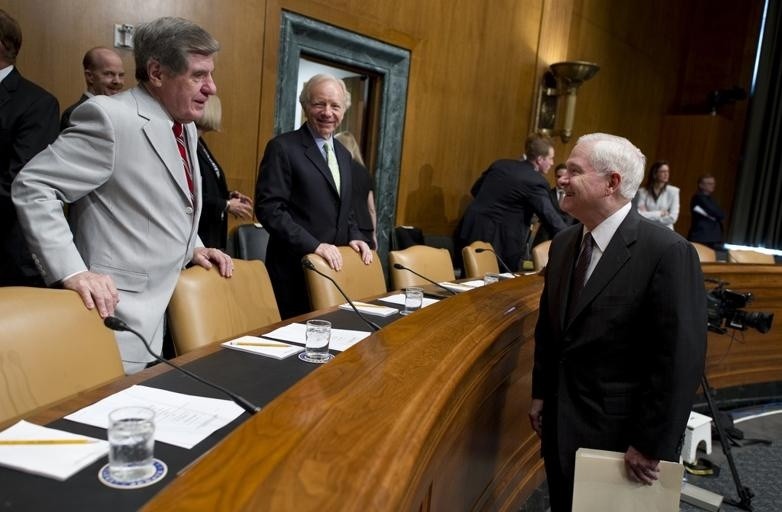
404 287 424 314
483 272 499 286
109 406 155 481
305 319 332 360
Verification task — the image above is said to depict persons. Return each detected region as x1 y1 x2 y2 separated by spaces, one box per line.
451 134 571 279
528 131 711 511
527 163 576 253
60 43 128 128
0 12 60 287
687 168 726 253
250 74 375 318
635 159 681 235
6 14 237 378
194 92 253 253
333 132 379 251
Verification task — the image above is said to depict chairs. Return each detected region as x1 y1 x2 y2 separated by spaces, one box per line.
530 239 553 273
304 245 389 307
689 240 717 265
390 245 458 292
0 287 126 421
391 224 425 252
726 249 776 266
462 241 501 277
232 223 270 267
166 260 284 356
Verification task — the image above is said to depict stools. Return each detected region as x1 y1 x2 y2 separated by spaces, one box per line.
680 410 714 464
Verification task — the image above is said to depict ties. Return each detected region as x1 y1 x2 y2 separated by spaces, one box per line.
565 233 593 324
324 137 343 201
558 191 563 206
170 120 195 208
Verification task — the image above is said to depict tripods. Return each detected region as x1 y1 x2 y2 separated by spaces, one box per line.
675 372 755 512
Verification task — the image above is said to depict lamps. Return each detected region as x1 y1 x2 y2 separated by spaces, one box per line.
533 60 602 142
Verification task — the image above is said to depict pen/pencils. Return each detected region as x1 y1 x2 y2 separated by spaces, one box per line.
349 303 386 308
443 283 474 287
0 439 100 446
230 341 293 347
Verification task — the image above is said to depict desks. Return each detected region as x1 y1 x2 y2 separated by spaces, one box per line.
1 255 782 512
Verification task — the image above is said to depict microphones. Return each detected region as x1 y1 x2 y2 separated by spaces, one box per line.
104 317 262 416
302 260 382 332
475 248 518 277
394 264 456 295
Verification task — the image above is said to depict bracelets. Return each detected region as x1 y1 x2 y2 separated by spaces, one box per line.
224 199 231 212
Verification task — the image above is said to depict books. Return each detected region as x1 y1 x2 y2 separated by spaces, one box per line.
0 419 109 482
433 280 475 294
337 300 398 317
219 333 303 361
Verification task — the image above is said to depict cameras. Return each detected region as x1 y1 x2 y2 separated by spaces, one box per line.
704 278 774 335
